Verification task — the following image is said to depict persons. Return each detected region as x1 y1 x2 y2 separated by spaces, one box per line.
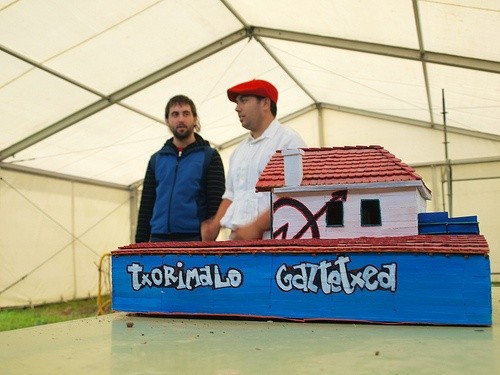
135 95 226 243
201 79 308 241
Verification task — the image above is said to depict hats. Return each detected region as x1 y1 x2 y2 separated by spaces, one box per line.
227 80 278 104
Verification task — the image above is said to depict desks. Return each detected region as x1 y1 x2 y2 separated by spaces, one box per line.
0 287 499 375
